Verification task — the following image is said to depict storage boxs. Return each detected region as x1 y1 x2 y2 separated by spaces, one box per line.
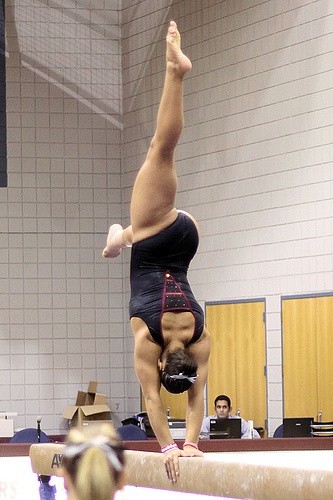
63 381 114 432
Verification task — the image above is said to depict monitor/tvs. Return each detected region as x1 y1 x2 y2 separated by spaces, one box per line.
282 417 314 438
209 418 241 440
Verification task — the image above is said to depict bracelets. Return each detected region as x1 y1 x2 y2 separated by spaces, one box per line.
161 443 177 454
183 442 198 449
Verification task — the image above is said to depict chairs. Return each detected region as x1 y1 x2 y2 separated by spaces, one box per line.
9 428 51 443
117 424 148 440
273 424 284 438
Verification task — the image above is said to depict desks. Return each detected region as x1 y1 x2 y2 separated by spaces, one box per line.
0 438 333 500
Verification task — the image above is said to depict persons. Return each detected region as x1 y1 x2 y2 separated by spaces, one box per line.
200 395 261 440
102 21 211 484
60 423 126 500
